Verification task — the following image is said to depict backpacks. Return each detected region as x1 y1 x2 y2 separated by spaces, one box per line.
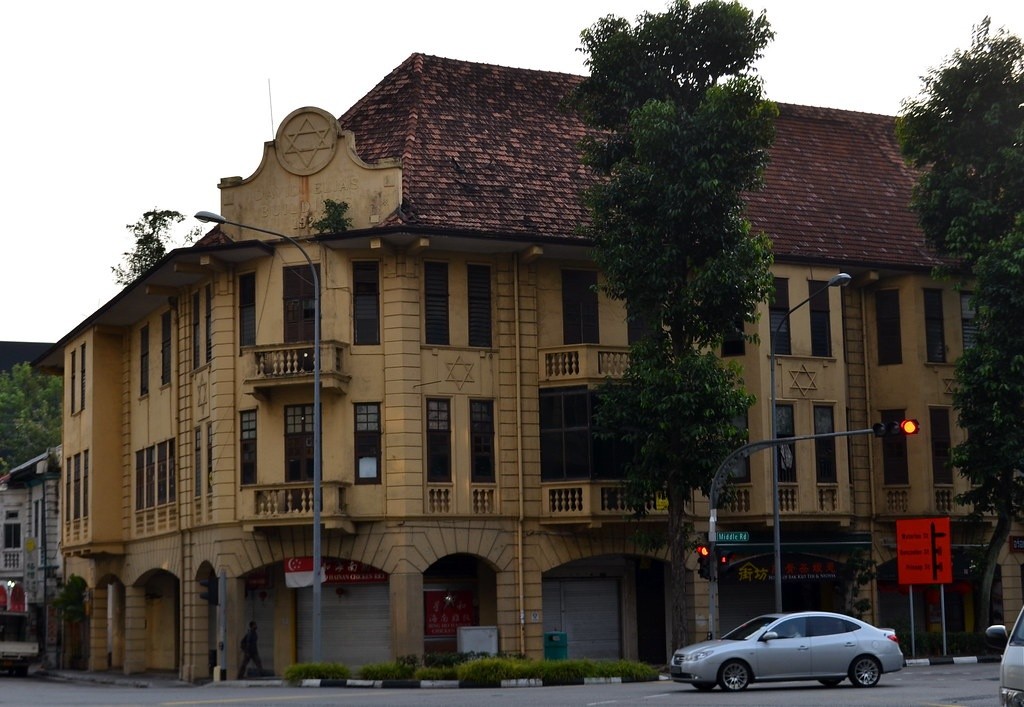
240 632 257 652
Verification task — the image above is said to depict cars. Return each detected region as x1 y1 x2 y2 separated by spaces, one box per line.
669 611 906 692
984 605 1024 707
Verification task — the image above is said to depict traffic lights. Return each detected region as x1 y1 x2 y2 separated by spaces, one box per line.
871 417 920 436
696 545 710 578
718 549 730 567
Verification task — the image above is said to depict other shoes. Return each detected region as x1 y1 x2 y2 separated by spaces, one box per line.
236 676 246 680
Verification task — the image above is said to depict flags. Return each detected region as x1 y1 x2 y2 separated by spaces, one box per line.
283 555 326 588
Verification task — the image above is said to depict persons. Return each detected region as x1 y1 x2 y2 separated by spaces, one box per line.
236 620 264 680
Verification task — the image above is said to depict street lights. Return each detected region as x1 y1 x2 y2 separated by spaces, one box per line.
765 273 850 610
195 211 325 671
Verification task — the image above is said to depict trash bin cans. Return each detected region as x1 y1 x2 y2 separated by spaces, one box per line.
544 631 569 660
